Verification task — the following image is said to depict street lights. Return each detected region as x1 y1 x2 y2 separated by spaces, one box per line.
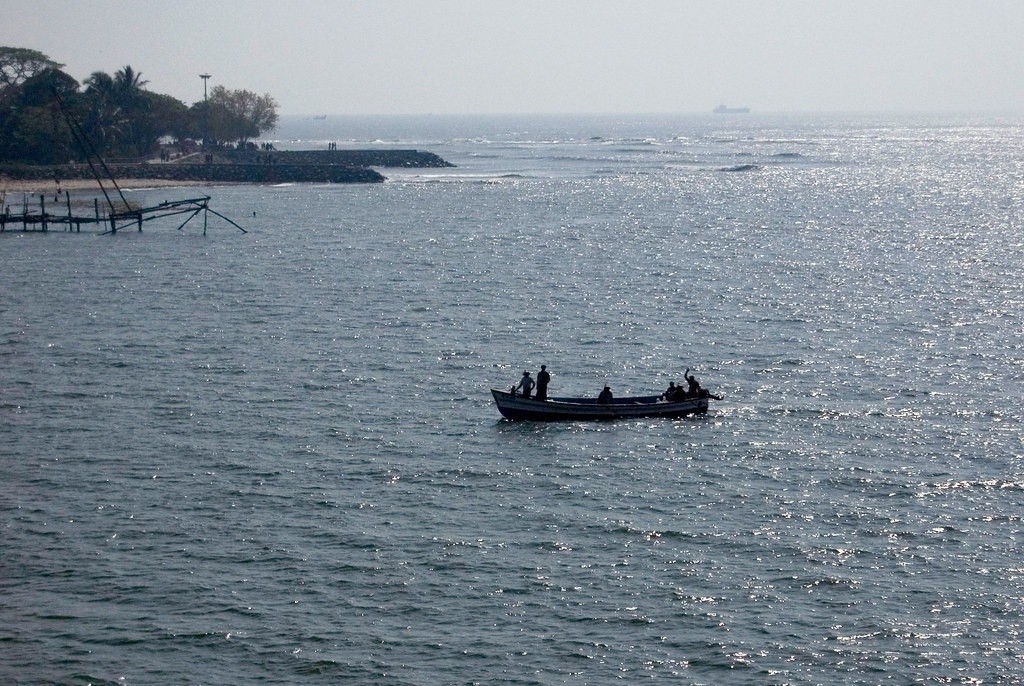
199 73 212 144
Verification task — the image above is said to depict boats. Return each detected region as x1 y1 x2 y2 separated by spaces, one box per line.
491 388 709 422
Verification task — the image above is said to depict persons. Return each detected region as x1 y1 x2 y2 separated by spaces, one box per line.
515 372 535 399
534 365 550 400
663 381 686 401
598 387 613 404
684 368 708 398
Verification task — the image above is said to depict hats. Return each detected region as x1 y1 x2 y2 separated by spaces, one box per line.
522 370 530 375
